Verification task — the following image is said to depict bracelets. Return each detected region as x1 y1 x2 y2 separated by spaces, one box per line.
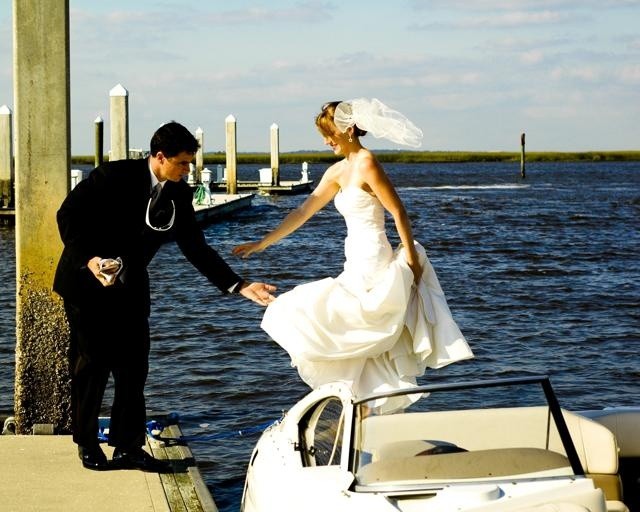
235 280 245 294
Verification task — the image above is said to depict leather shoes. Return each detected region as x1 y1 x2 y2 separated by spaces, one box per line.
111 448 171 472
78 445 109 470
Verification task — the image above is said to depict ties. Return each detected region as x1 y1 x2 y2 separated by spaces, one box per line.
151 185 160 205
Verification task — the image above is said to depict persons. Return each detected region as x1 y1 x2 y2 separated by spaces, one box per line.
54 121 281 475
231 98 477 418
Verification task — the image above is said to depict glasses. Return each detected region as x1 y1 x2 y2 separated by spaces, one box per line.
144 198 176 232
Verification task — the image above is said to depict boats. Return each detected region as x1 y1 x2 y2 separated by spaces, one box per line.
241 380 640 512
